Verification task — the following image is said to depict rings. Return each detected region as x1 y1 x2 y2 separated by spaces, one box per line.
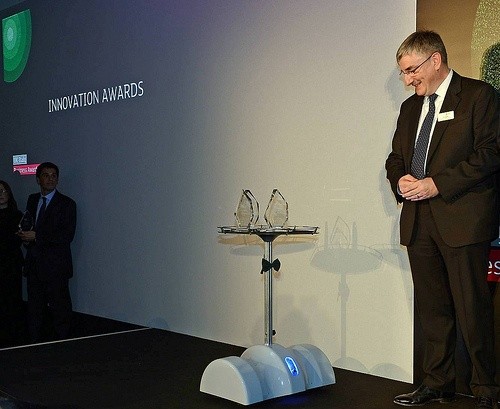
417 194 420 198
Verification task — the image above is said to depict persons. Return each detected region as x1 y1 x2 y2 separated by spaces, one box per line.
0 179 24 349
15 162 77 367
384 29 499 409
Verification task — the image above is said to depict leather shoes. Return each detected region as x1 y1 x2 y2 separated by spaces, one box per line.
476 396 496 409
393 384 456 407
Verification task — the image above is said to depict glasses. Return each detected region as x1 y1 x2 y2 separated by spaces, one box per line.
399 53 432 77
0 189 7 192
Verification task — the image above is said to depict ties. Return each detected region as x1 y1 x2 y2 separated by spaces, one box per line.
411 93 438 179
37 196 48 225
